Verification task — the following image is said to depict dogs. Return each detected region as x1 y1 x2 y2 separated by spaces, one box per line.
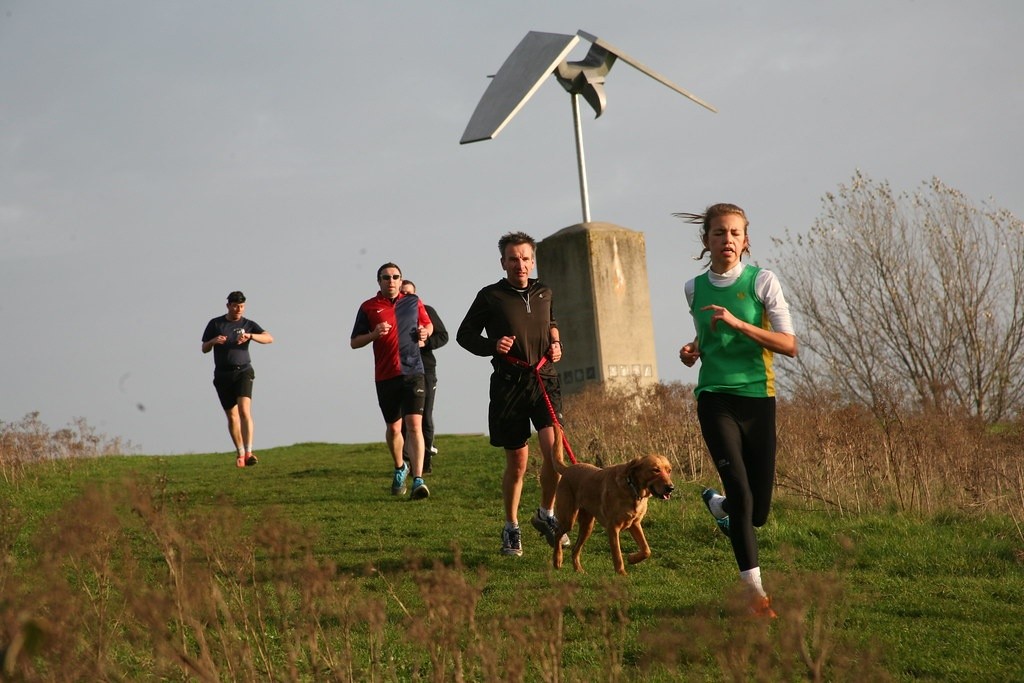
550 422 676 575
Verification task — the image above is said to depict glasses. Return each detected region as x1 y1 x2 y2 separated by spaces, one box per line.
380 275 401 280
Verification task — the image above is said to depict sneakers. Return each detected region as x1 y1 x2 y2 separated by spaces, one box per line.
410 478 430 500
748 595 779 624
530 508 571 550
501 526 523 558
701 487 729 538
236 452 258 468
391 460 410 495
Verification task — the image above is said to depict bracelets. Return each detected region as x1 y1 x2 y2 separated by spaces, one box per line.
552 340 563 352
250 334 252 339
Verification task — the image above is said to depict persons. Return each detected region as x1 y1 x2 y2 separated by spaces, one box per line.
351 262 449 498
671 203 798 618
456 232 570 556
201 291 273 467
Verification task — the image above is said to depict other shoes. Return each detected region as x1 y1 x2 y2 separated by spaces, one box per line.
409 463 432 477
431 445 439 457
